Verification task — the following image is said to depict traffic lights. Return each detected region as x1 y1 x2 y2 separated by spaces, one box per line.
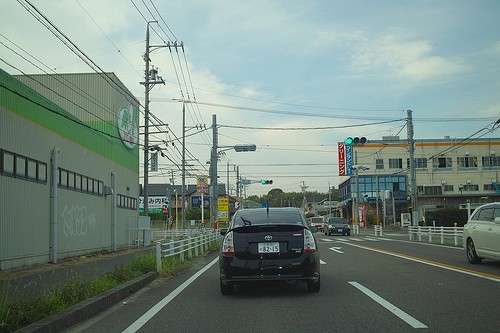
261 180 273 186
344 137 367 145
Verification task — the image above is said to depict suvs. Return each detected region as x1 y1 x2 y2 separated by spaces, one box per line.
309 217 325 233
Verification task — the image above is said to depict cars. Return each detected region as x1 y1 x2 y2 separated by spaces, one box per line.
462 203 500 266
218 208 321 296
323 217 350 237
